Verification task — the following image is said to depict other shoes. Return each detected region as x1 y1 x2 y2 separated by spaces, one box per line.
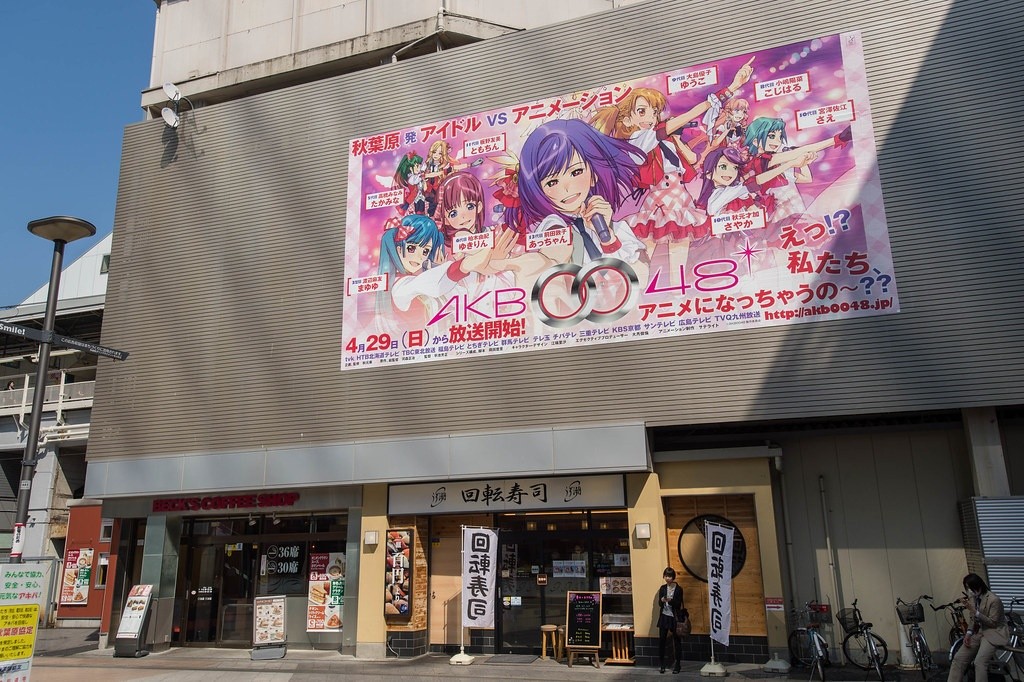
660 665 666 674
672 664 681 674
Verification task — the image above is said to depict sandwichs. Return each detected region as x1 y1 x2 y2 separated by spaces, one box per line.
310 585 328 604
66 571 76 585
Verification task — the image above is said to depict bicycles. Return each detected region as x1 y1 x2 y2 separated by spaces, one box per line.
894 594 935 680
788 600 830 682
835 597 888 682
930 591 1024 682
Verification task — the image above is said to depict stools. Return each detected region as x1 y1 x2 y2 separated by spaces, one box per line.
540 624 558 660
557 626 570 663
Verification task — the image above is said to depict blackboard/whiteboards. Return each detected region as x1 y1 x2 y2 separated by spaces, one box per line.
566 591 603 648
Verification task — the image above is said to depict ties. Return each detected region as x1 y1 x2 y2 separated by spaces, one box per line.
977 596 981 611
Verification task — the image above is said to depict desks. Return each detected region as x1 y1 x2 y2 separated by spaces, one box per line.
569 649 600 668
602 624 635 663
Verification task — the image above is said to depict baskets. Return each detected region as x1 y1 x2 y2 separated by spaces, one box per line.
896 603 925 625
786 608 806 630
835 608 857 633
1005 612 1023 632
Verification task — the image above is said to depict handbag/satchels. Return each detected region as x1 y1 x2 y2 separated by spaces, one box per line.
676 616 692 636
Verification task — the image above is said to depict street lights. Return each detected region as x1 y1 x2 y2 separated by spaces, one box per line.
7 216 97 565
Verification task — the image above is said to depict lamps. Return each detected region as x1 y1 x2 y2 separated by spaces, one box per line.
547 522 557 534
581 520 588 530
600 520 608 533
249 513 256 526
618 540 629 549
272 511 281 526
527 520 537 534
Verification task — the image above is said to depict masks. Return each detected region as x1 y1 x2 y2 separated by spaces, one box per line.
969 588 979 597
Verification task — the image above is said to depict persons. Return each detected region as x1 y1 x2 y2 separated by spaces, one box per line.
656 567 684 675
2 380 15 406
946 573 1012 682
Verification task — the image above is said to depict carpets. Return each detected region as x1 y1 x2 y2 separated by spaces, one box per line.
483 655 539 664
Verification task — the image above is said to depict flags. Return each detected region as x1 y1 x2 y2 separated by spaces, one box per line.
707 523 734 647
462 527 498 630
